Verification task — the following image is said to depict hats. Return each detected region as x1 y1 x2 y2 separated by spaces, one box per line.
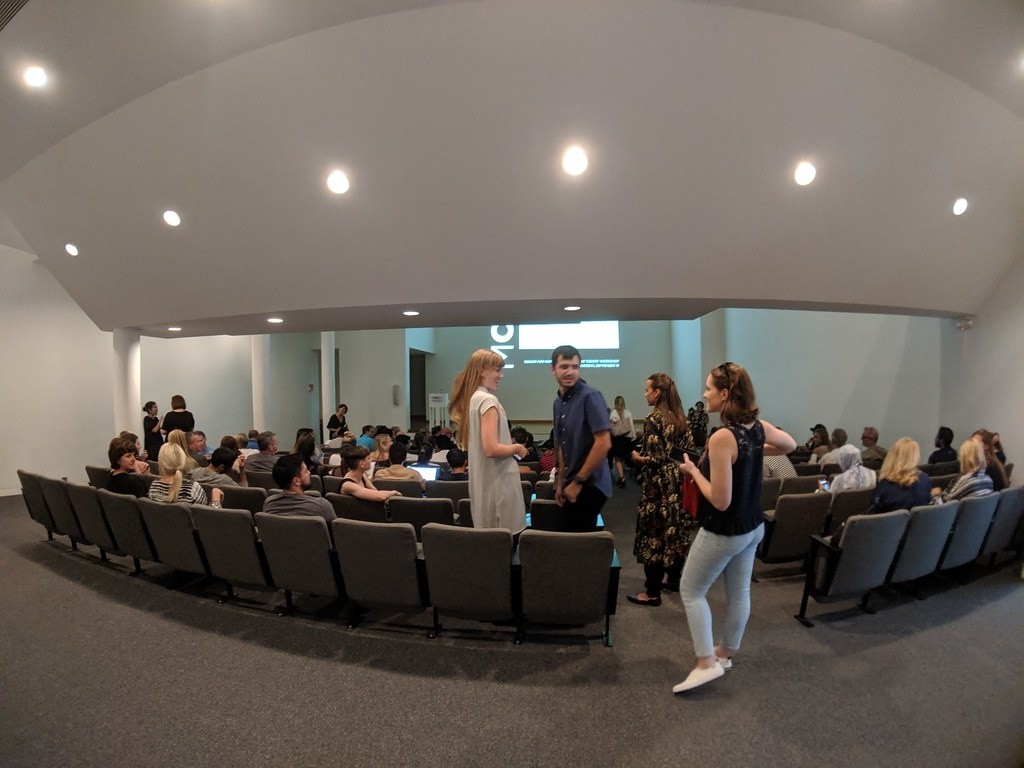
810 424 826 433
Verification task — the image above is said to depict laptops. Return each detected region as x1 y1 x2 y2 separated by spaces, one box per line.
406 465 440 481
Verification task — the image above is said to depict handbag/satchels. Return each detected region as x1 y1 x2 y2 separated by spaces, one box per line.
682 444 709 518
611 417 631 437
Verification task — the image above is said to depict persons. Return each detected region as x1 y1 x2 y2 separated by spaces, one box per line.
510 426 556 483
607 396 644 489
551 346 613 531
119 395 323 487
149 443 223 511
106 438 148 499
760 455 797 480
671 363 796 693
628 373 695 606
450 348 528 551
327 404 468 500
690 401 718 448
807 424 1010 513
264 453 336 522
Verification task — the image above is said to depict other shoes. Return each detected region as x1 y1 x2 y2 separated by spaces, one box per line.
713 646 732 671
627 593 662 607
617 476 626 488
661 578 680 592
672 661 724 693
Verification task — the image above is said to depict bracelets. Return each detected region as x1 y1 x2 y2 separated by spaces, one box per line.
574 473 587 485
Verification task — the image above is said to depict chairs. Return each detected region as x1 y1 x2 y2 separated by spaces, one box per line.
757 447 1024 628
16 438 620 647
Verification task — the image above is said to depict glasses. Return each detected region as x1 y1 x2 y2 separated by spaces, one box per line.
862 433 871 440
718 362 733 381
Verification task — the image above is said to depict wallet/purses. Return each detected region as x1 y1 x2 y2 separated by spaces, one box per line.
669 444 701 467
